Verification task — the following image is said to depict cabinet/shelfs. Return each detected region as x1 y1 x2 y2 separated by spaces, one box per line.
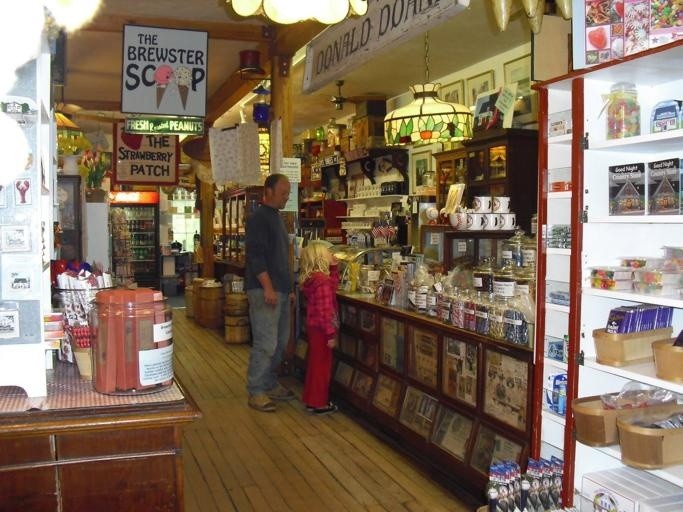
529 40 683 510
0 51 56 402
290 283 534 511
432 134 538 224
296 180 347 244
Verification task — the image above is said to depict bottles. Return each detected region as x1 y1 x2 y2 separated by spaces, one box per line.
515 273 535 302
131 207 136 219
500 239 520 268
135 220 139 232
130 247 157 260
140 207 143 219
531 213 537 238
127 221 131 232
472 256 493 291
519 240 535 268
135 208 139 219
492 272 516 301
143 207 155 218
143 220 155 232
124 206 131 220
130 233 156 246
131 220 135 232
139 220 143 231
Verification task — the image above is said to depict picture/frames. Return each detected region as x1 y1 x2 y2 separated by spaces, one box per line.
466 70 495 106
503 54 529 85
438 80 464 106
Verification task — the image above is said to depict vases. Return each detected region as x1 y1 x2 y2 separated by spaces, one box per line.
85 186 107 201
57 152 85 176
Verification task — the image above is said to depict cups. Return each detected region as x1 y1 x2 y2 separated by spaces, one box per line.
467 214 488 230
485 213 500 230
448 213 467 230
501 214 516 230
493 197 510 213
472 197 492 212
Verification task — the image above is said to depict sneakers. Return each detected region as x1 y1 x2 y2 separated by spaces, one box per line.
314 405 340 417
248 393 277 411
267 380 295 399
306 402 335 413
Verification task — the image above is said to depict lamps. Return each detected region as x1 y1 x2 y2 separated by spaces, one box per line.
224 0 368 25
383 30 474 147
252 84 271 124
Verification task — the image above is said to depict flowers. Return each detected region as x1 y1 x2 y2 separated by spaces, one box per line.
83 150 110 187
58 133 91 153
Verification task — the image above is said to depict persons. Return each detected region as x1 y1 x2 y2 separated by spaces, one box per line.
298 240 340 415
244 174 295 412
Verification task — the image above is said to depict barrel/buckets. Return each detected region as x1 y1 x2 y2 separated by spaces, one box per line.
224 314 252 344
198 285 225 329
192 278 206 324
185 286 195 318
225 294 249 316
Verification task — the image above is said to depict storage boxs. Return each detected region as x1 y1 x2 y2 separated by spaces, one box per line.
354 99 385 120
592 326 672 367
615 404 682 470
567 389 676 448
652 339 683 382
351 117 384 149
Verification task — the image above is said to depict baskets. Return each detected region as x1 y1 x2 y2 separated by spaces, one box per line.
66 328 94 380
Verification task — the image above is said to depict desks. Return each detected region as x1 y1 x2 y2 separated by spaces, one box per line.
1 308 204 511
